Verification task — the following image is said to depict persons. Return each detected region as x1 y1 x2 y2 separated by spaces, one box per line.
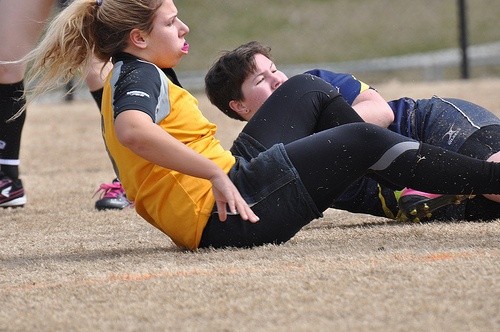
0 0 137 212
200 40 500 225
4 0 500 254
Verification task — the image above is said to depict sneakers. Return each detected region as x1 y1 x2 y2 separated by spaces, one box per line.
0 172 26 209
95 179 130 211
398 187 476 222
